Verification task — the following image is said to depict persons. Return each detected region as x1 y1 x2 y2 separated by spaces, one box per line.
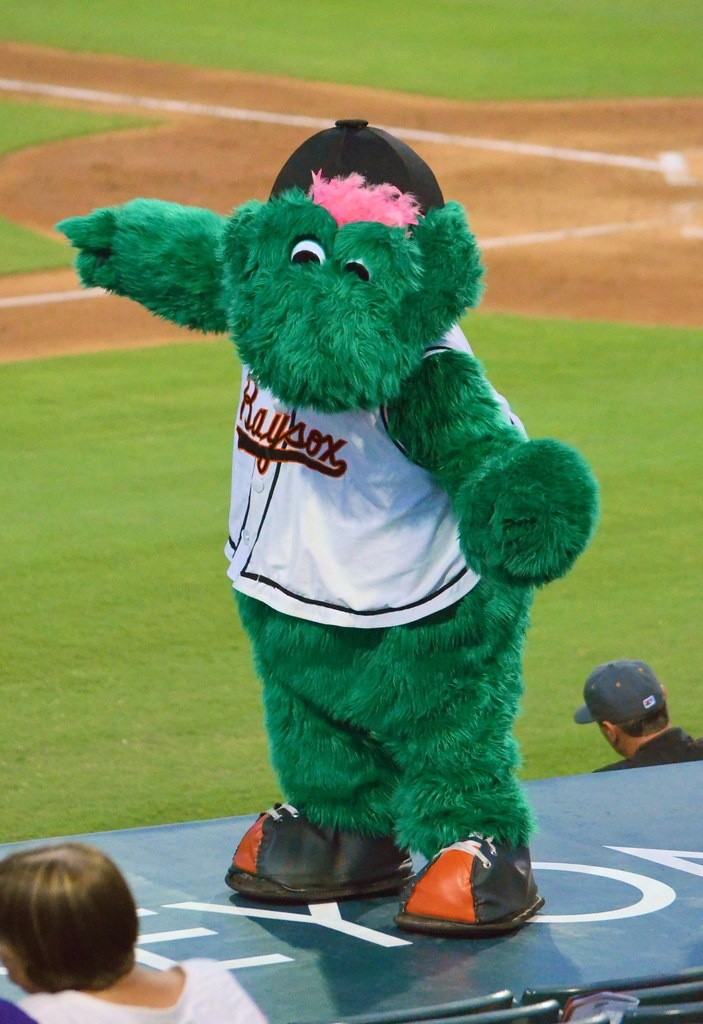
573 659 703 775
0 844 272 1024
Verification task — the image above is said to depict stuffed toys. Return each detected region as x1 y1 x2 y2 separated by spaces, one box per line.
63 118 596 938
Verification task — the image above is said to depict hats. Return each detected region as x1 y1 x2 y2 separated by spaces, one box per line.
573 656 667 726
